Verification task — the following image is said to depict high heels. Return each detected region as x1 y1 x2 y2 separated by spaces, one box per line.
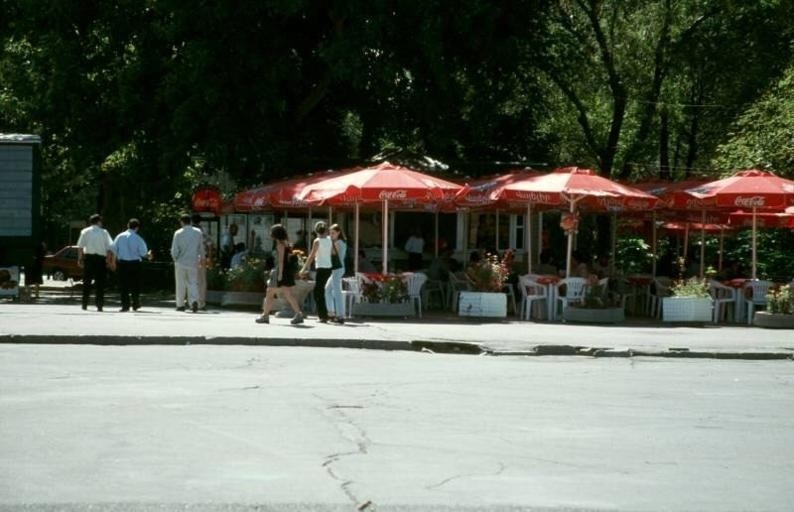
317 315 345 324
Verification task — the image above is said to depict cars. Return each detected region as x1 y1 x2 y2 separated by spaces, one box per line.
40 245 85 282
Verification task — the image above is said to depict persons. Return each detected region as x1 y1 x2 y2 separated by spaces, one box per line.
171 214 747 325
111 218 153 312
21 238 48 298
78 214 113 311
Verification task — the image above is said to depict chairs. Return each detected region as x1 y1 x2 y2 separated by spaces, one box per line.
299 249 779 327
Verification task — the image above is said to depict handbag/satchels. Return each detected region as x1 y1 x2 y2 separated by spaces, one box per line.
286 252 301 273
331 240 343 270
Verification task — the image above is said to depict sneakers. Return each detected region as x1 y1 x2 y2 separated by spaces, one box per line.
176 307 185 311
291 315 303 324
255 314 269 324
191 301 199 314
